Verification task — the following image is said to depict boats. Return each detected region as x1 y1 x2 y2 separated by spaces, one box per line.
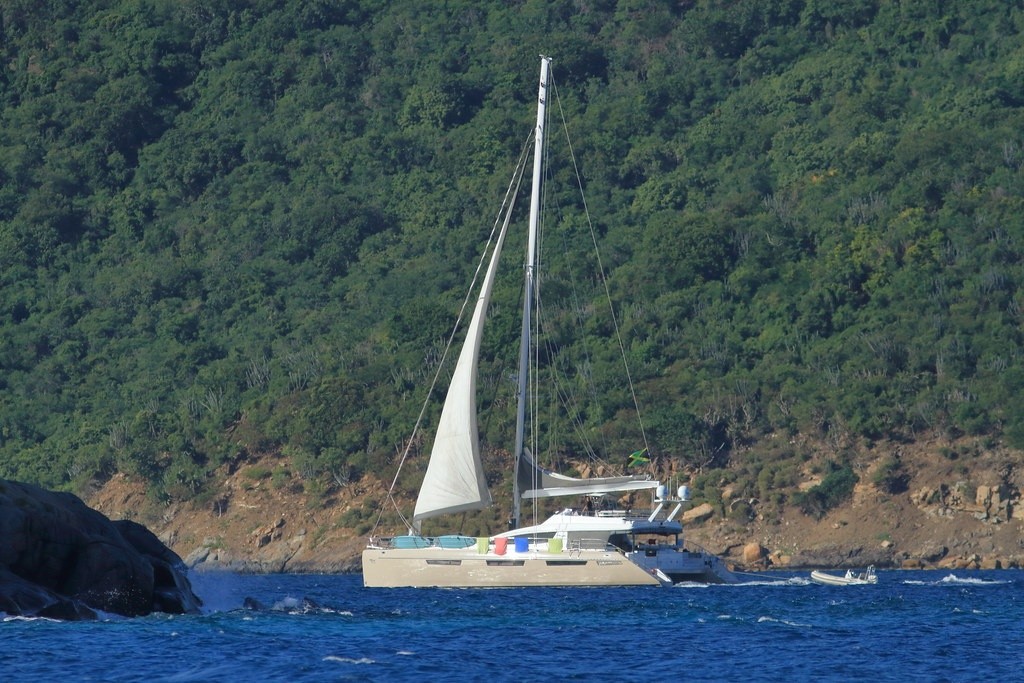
811 564 879 588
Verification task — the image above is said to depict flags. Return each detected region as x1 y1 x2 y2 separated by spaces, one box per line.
628 447 651 468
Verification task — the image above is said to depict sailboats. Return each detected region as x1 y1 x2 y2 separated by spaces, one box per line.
360 49 748 590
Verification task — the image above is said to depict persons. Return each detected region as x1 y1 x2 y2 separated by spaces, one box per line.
586 497 592 516
